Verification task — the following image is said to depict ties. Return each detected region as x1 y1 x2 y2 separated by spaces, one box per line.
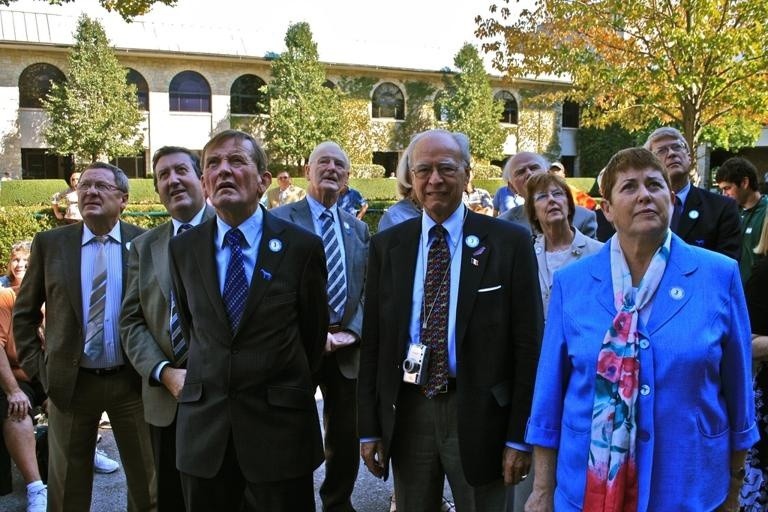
222 229 249 336
83 236 107 361
321 210 347 312
170 227 188 368
419 227 450 398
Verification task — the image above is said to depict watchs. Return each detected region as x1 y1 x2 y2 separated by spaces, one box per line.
729 466 748 483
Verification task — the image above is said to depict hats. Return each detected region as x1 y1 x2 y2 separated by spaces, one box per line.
550 162 564 172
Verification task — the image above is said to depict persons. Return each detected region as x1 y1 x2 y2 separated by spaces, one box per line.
10 162 158 511
644 127 744 266
117 145 216 512
166 128 330 511
463 151 617 326
0 240 121 511
738 206 767 511
49 171 84 226
523 145 761 512
267 169 307 209
377 147 424 233
715 156 768 249
336 186 369 220
357 128 545 512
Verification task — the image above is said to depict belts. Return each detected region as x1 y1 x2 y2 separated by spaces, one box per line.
414 377 456 394
80 368 121 374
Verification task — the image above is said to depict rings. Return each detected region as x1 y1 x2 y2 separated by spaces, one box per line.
520 472 529 481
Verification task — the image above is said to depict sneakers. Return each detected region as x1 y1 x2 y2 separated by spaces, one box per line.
94 450 119 473
25 485 47 512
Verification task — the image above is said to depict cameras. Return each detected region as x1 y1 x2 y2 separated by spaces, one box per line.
402 344 430 385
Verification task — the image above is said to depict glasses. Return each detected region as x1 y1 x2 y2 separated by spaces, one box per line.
13 242 34 250
654 142 686 156
75 182 119 191
411 164 465 179
